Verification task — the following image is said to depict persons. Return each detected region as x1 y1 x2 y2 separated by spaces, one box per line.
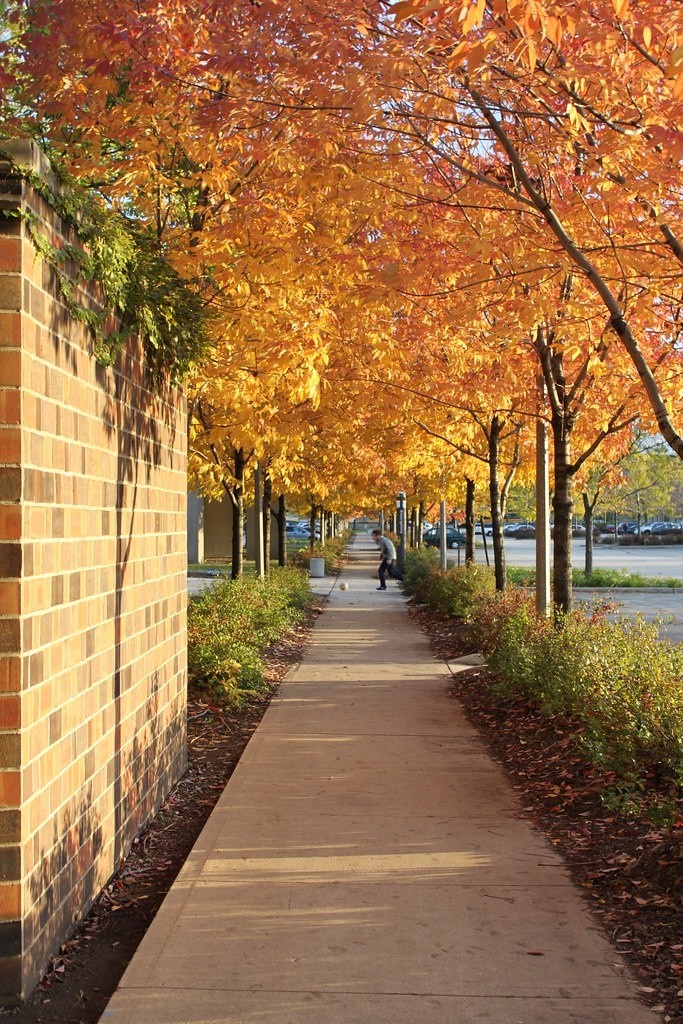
371 530 404 590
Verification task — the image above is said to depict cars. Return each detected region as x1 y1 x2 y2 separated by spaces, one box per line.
423 526 466 550
402 518 683 538
285 521 321 534
286 526 321 542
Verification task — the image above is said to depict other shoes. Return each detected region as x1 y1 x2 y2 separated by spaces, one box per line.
376 586 386 590
